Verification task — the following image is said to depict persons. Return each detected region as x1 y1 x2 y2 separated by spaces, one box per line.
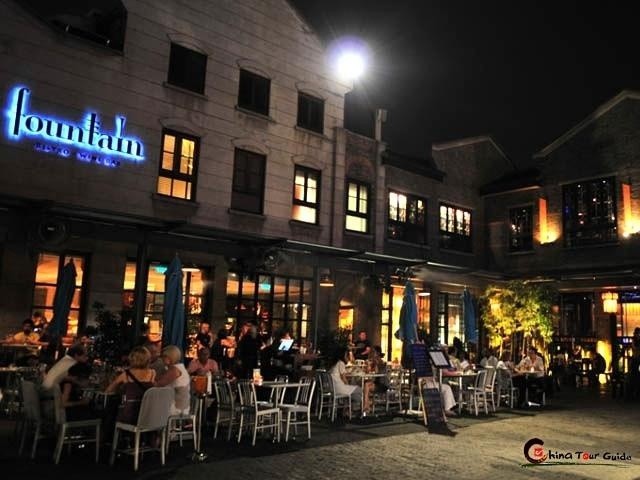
1 312 390 451
417 327 640 416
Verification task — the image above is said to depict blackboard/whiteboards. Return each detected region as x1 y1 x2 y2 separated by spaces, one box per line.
409 343 444 424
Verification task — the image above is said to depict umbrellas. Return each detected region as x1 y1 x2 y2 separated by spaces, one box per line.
45 258 77 362
395 279 419 410
160 251 186 364
460 285 478 344
